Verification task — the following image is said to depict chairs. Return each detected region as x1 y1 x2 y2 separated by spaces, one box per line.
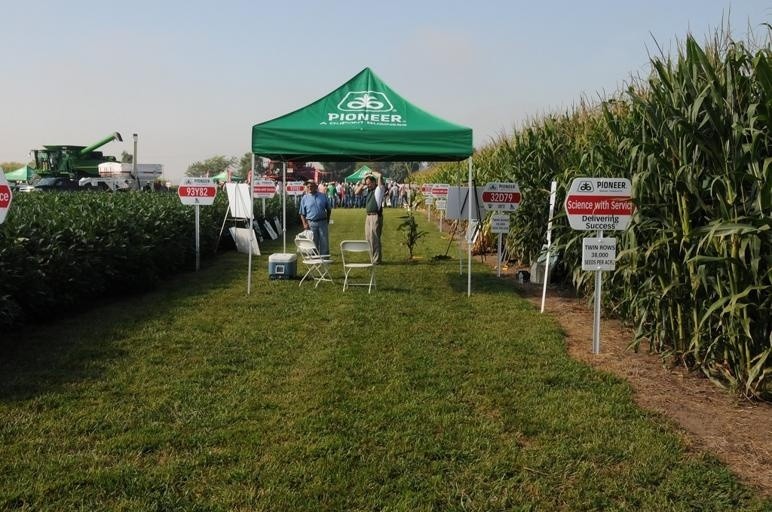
294 239 380 294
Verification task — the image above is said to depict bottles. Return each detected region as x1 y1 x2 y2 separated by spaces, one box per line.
518 272 524 292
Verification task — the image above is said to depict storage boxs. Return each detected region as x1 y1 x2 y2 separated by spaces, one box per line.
268 252 297 280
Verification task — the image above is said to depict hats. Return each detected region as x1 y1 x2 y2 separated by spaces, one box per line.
303 179 315 185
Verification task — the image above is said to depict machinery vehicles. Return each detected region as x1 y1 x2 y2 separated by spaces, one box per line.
31 132 125 177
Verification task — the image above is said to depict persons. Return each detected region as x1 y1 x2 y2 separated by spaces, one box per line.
317 180 408 208
298 179 331 260
354 175 385 265
